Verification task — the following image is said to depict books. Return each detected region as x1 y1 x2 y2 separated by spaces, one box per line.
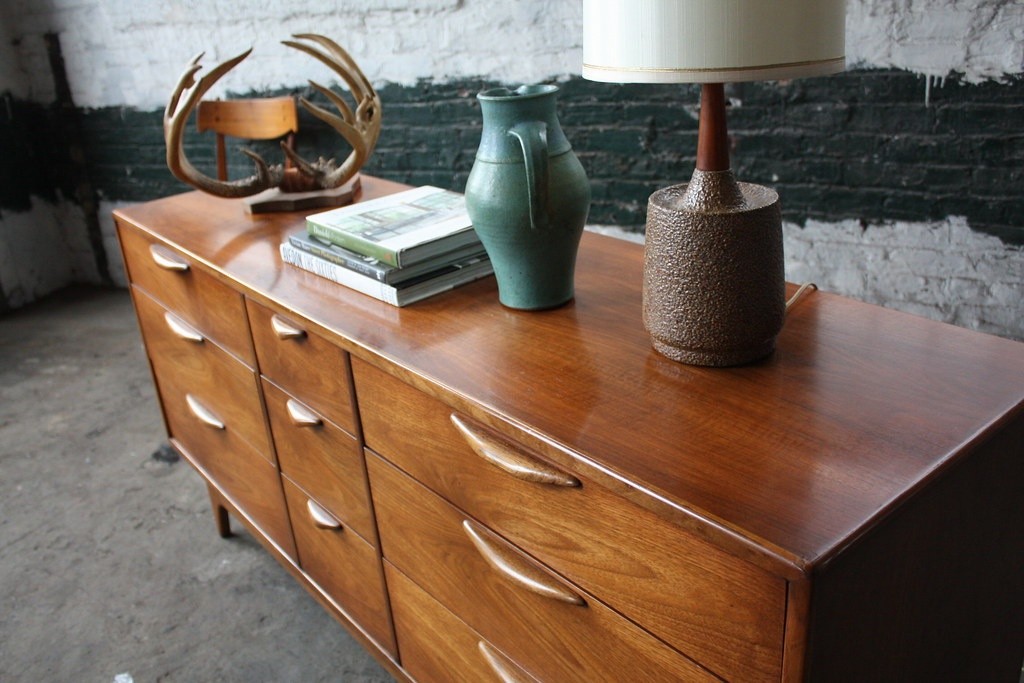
277 183 494 308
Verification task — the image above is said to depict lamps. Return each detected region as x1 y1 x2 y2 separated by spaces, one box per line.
581 1 846 366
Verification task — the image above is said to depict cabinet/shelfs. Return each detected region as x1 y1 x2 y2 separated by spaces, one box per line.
111 163 1024 682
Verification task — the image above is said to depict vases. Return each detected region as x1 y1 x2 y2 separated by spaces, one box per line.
463 84 591 312
